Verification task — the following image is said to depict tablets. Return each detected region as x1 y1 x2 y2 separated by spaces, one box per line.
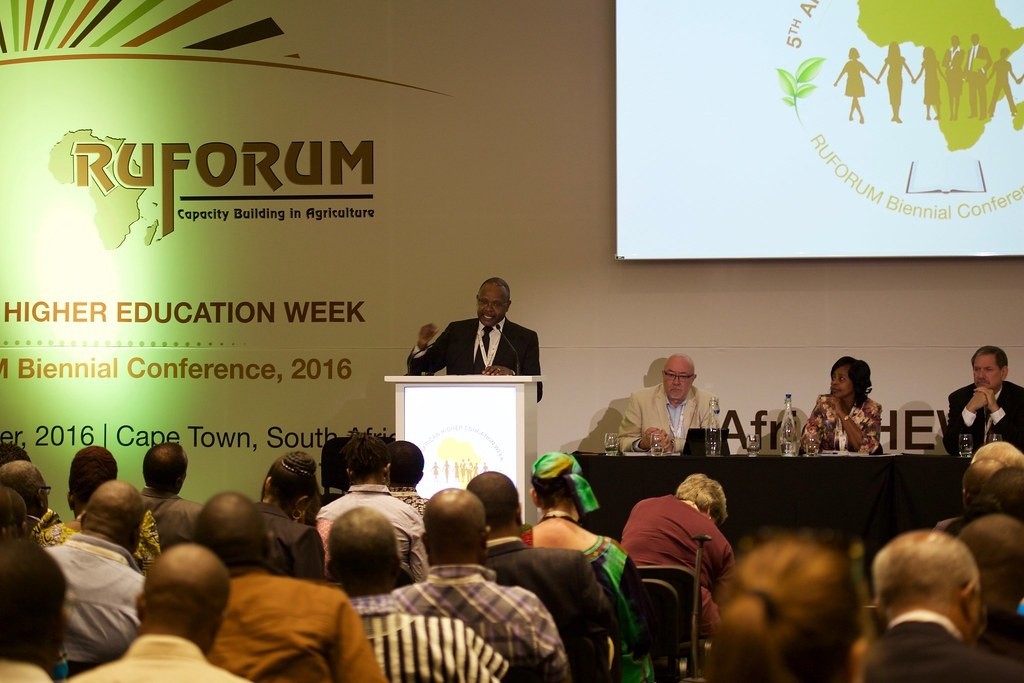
682 427 731 455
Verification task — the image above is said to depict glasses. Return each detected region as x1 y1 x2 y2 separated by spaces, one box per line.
665 373 693 382
479 298 506 308
41 486 51 495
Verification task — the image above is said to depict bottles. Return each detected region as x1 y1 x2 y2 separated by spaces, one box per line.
705 396 721 456
779 393 795 453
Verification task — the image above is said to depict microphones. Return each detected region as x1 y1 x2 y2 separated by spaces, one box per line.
406 332 450 376
496 324 521 375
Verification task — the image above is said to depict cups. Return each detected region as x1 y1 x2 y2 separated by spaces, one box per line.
747 434 760 457
988 434 1002 443
604 432 619 455
806 436 818 455
958 433 972 457
650 434 664 456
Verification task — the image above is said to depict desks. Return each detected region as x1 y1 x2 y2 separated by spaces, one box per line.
571 451 972 586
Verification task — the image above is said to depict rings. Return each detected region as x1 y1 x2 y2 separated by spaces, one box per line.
497 368 501 372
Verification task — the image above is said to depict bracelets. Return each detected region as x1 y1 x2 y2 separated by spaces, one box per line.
841 415 850 422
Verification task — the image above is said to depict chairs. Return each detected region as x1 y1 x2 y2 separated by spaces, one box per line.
634 564 711 683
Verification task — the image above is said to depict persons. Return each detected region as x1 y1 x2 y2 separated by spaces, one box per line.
942 346 1024 455
704 527 875 683
0 442 388 683
521 450 657 683
406 276 543 403
253 431 622 683
800 355 882 455
614 353 720 452
620 474 734 636
861 441 1024 683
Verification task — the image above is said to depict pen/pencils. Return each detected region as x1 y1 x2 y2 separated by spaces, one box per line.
574 453 606 456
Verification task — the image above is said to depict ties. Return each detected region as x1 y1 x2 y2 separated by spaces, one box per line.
482 326 494 357
985 408 991 426
